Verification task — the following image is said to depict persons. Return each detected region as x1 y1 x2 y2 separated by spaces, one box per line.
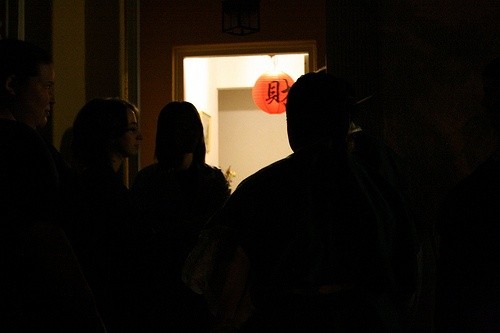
437 59 500 333
0 39 228 333
210 72 422 333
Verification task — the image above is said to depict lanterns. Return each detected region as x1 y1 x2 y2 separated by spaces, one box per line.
252 72 295 115
